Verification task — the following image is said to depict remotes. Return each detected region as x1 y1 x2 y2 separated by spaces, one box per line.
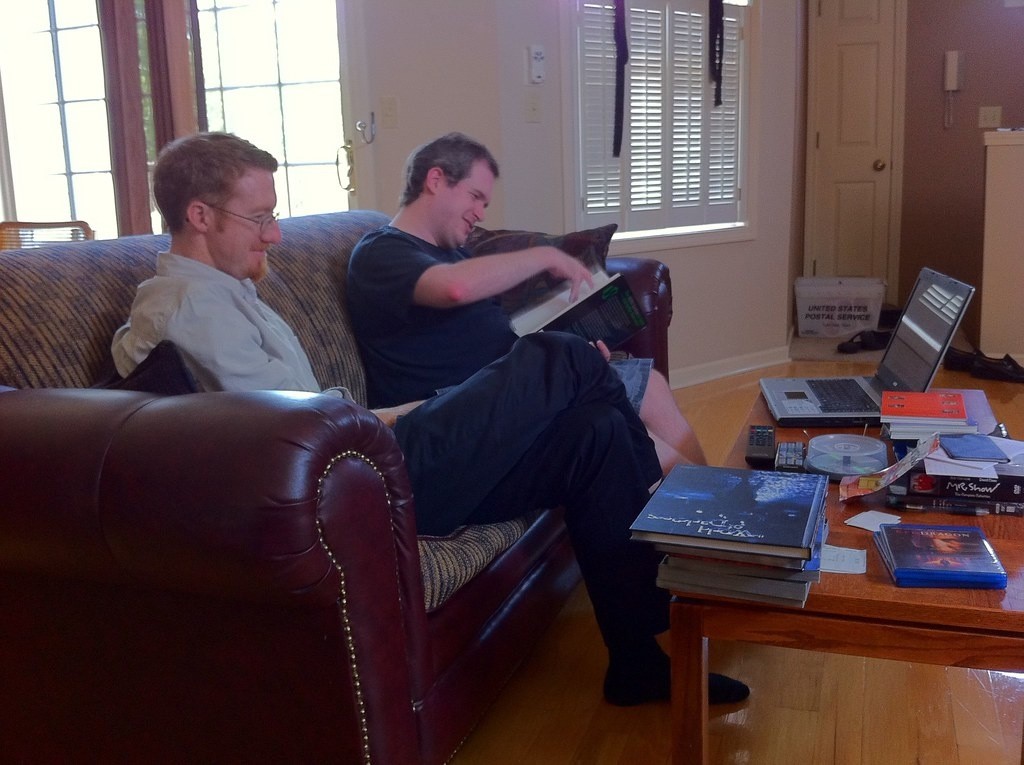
774 442 804 472
744 425 775 467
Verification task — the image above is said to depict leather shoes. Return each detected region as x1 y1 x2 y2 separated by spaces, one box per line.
970 350 1024 383
944 346 984 371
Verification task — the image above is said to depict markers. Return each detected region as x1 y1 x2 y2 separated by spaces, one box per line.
993 127 1015 131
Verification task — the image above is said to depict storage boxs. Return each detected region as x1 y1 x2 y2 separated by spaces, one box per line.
794 277 887 338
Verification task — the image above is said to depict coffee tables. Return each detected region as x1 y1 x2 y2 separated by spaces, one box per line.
670 391 1023 765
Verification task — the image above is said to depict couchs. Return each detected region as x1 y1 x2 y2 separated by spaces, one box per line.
0 210 673 765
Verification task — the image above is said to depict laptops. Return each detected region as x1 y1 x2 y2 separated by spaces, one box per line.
758 266 975 428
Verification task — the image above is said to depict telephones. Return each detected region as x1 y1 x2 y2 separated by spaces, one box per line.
944 50 959 92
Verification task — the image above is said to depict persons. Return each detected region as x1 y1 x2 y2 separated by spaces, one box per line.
109 131 749 706
345 133 708 476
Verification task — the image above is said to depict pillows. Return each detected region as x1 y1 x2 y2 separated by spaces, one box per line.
464 224 619 314
102 338 206 396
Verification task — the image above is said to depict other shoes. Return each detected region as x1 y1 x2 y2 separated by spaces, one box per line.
861 330 892 349
604 659 750 707
837 332 865 354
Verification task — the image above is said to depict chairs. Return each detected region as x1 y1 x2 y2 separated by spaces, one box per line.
0 220 96 253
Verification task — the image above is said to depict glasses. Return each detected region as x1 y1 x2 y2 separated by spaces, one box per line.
187 202 282 229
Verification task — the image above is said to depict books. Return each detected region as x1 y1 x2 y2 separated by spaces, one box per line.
879 390 977 440
509 269 647 351
626 462 828 607
886 433 1024 517
874 518 1008 589
